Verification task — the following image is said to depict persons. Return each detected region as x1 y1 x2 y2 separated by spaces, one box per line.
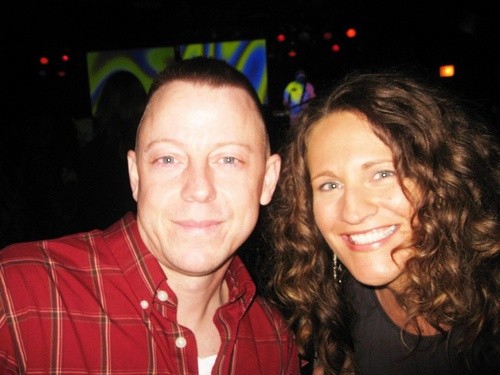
0 57 302 375
77 71 149 232
254 71 500 375
